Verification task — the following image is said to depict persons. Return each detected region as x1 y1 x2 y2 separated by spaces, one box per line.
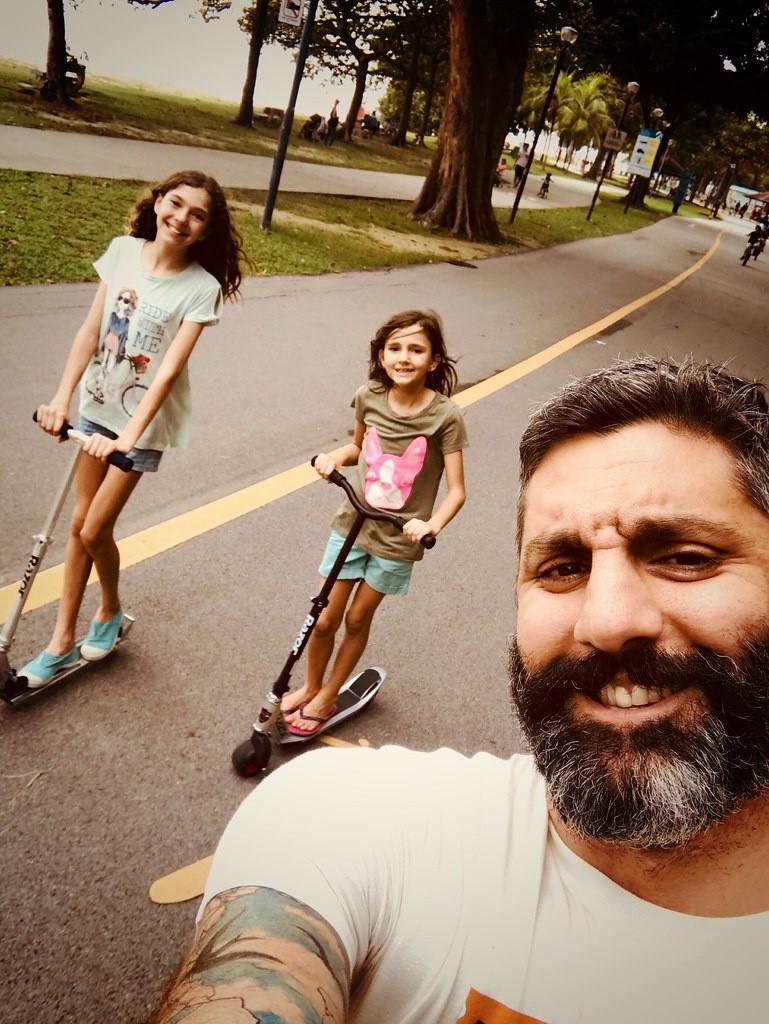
156 355 769 1024
325 97 552 196
731 198 769 259
21 169 253 685
259 310 468 736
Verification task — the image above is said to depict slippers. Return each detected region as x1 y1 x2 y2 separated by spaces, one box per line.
258 702 338 735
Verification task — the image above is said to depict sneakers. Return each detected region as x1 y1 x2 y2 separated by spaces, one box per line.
79 602 124 660
19 647 80 688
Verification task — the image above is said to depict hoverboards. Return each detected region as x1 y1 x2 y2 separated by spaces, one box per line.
231 453 388 777
2 412 137 705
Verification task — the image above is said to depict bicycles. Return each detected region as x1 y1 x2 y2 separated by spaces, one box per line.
493 171 503 188
741 236 760 266
539 178 553 199
86 345 148 417
753 237 768 260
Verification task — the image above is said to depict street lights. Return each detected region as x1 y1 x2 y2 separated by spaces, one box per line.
509 26 578 224
649 138 674 198
624 107 664 215
712 163 737 218
586 81 639 221
